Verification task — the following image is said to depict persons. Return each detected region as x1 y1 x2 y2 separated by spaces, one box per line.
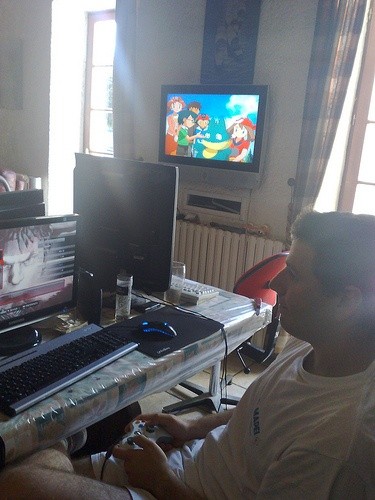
1 207 375 499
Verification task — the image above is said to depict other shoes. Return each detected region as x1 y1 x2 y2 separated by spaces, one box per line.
59 428 87 454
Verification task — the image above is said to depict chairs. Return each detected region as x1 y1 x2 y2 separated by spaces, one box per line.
161 250 294 419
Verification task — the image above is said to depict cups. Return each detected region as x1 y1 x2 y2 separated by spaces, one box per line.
115 272 133 322
163 260 186 305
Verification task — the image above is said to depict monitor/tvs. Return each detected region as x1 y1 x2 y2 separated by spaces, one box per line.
161 83 270 189
0 214 80 354
73 152 179 289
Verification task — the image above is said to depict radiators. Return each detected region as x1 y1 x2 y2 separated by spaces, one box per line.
174 222 286 349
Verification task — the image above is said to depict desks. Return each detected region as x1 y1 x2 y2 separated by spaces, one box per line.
0 275 271 460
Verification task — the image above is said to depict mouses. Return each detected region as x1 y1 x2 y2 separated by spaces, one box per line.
141 321 178 339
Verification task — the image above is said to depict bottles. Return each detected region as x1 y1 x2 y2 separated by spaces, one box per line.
0 170 17 192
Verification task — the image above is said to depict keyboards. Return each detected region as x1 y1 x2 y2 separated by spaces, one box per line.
0 323 140 415
168 275 220 305
